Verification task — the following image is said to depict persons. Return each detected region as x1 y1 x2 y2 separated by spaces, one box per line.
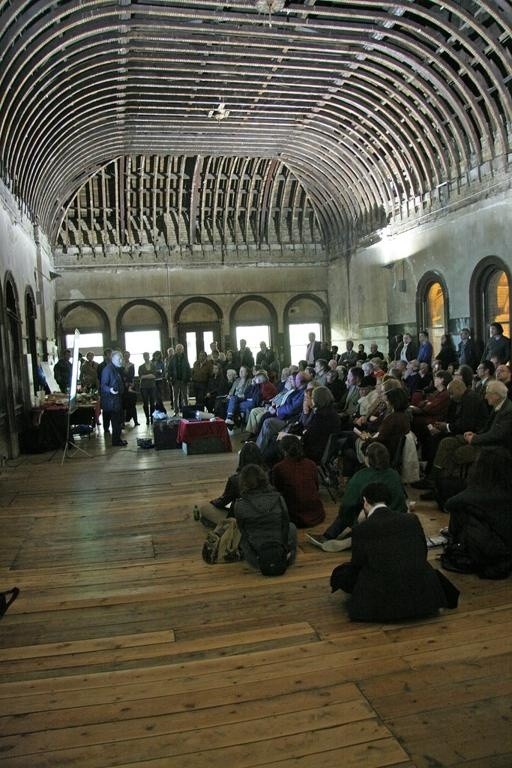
329 482 460 623
192 324 512 580
54 342 189 446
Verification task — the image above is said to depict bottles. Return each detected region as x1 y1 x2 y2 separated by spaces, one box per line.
81 393 87 404
196 409 201 421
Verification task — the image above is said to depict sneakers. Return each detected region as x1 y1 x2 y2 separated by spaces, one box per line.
112 440 127 446
410 477 435 500
240 437 257 443
225 418 236 425
304 533 352 553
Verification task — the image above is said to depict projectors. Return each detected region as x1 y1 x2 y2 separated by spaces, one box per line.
195 411 214 421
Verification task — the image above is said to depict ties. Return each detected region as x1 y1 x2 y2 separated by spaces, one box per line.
306 343 312 360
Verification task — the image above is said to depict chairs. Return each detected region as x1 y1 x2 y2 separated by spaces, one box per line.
315 431 347 502
235 410 246 432
394 431 418 499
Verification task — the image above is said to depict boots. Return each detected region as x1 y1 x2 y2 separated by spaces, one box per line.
144 405 153 425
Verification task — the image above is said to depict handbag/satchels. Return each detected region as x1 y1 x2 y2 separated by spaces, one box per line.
317 462 341 505
257 542 288 576
441 541 478 573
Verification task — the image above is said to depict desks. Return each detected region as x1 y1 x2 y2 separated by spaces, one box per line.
154 416 182 451
44 393 101 451
177 417 233 456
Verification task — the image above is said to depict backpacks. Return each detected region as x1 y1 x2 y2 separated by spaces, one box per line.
202 517 244 564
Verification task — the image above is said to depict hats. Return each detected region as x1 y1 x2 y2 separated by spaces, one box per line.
359 376 377 387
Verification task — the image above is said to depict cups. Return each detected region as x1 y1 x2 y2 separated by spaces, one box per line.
37 391 46 401
409 500 417 511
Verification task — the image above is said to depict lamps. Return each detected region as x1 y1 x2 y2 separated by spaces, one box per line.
255 0 286 29
208 103 229 126
33 268 61 282
384 260 403 269
